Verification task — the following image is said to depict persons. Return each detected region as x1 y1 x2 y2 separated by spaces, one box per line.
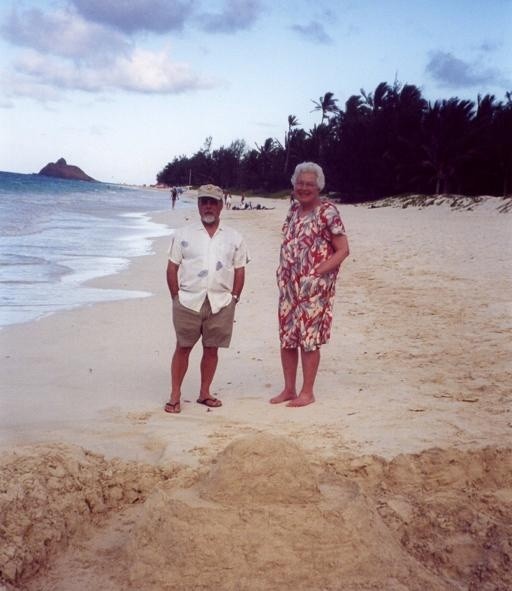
270 159 350 405
162 184 252 414
168 185 183 207
290 189 295 204
224 190 275 210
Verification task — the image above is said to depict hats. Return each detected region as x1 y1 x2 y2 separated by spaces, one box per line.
196 184 225 202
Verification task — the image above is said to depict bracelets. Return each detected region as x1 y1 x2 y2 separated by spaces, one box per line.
233 294 240 301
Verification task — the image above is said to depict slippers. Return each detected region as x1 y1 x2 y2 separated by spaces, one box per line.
164 401 181 414
197 397 224 409
